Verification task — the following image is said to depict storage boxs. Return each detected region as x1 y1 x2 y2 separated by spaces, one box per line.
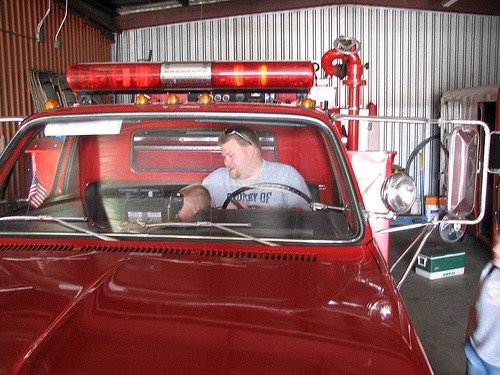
415 246 466 281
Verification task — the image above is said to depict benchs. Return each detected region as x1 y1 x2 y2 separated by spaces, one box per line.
85 180 319 232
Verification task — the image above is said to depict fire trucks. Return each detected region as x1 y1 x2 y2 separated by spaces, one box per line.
0 61 436 375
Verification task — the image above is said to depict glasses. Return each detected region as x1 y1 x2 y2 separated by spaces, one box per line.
221 128 252 146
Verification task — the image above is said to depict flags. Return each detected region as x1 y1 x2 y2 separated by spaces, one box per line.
26 170 48 208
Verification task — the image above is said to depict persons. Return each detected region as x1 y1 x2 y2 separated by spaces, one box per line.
461 228 500 375
174 184 211 222
202 127 314 211
